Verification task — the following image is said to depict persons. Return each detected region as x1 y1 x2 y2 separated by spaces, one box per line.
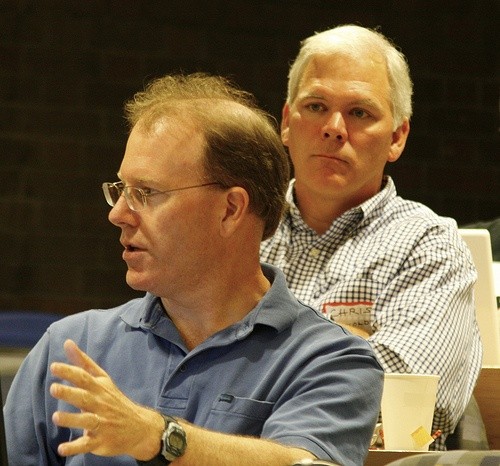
3 72 385 466
258 24 462 451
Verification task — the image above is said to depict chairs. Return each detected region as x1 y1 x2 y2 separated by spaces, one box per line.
0 312 60 369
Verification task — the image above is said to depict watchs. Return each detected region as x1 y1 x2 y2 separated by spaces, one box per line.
135 412 187 466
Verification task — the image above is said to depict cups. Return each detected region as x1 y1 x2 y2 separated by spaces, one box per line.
380 374 440 450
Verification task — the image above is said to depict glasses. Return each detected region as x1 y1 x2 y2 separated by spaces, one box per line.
101 179 228 213
